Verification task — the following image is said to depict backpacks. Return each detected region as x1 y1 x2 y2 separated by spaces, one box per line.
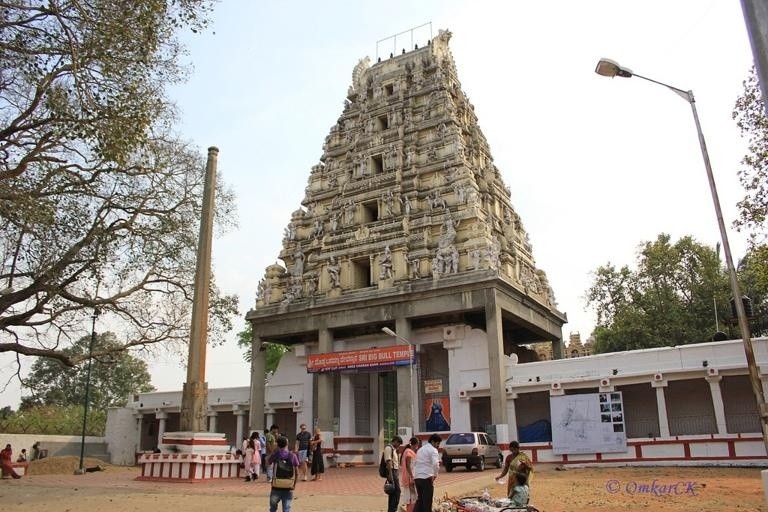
272 451 296 489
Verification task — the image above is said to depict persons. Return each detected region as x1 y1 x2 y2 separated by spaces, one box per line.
496 441 534 499
0 444 21 479
33 445 42 459
508 475 530 506
242 424 323 483
17 449 26 462
426 399 450 431
265 436 300 512
380 436 402 512
413 434 442 512
399 437 420 512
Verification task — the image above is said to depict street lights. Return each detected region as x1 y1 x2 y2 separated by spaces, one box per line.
74 302 102 475
381 324 417 441
595 58 767 460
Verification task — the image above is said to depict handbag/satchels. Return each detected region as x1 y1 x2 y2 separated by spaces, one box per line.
384 480 395 494
380 453 389 478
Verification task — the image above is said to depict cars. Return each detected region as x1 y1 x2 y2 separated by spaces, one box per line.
443 431 504 472
